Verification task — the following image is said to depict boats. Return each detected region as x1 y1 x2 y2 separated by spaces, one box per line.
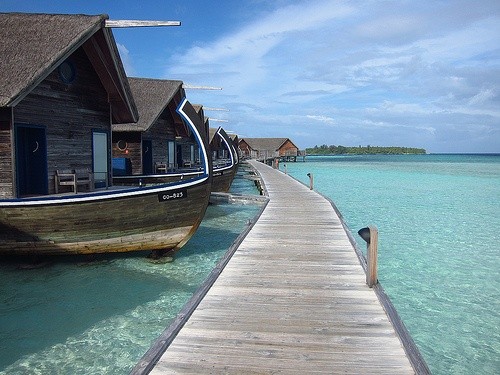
177 137 239 172
0 99 213 269
110 127 237 193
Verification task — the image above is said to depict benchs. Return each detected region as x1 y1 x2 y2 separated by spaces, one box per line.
54 167 94 194
